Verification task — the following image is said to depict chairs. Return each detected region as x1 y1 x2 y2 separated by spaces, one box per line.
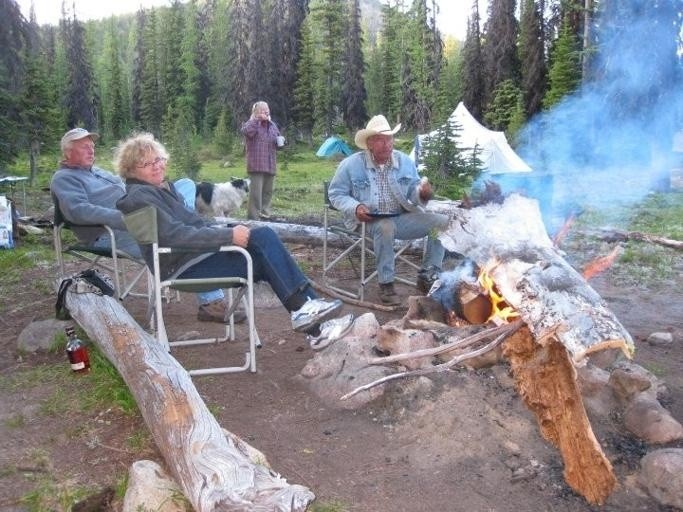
151 211 260 374
52 186 150 301
321 180 428 303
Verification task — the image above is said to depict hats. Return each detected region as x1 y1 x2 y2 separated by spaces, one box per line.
62 128 99 142
354 115 401 150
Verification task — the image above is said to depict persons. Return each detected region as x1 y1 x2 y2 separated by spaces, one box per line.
241 101 286 220
114 131 355 352
326 114 449 306
50 128 246 323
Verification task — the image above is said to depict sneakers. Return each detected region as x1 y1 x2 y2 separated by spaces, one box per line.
416 277 436 290
310 313 354 350
290 295 343 333
377 283 402 306
197 300 246 323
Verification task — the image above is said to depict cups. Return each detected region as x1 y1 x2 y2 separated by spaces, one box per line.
275 136 284 148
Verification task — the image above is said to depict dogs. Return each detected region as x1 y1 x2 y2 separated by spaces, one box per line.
196 176 252 217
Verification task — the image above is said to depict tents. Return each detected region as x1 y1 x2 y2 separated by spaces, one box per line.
316 136 352 158
408 101 533 174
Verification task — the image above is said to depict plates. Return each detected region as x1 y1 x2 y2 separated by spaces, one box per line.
366 210 399 218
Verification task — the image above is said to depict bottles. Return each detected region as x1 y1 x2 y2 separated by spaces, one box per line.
62 325 89 372
416 176 428 191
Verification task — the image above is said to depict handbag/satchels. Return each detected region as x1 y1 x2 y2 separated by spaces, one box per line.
84 267 115 296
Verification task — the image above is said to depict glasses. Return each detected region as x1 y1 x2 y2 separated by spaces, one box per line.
139 156 162 169
